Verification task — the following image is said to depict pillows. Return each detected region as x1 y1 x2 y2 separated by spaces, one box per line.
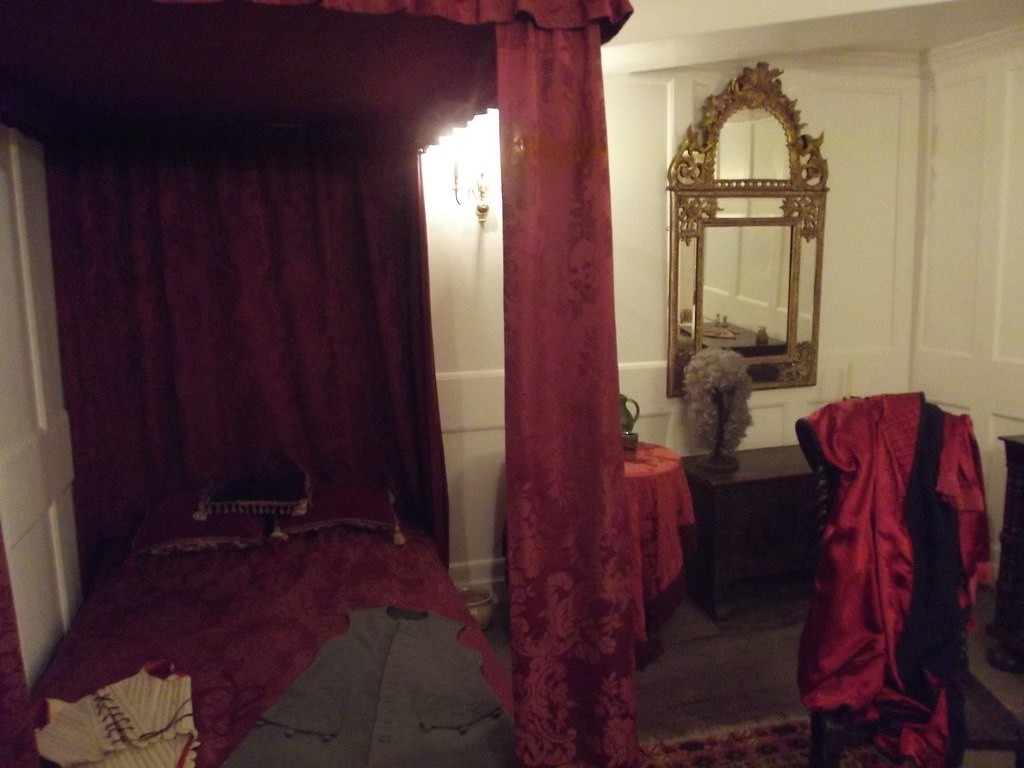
194 457 314 519
270 471 407 546
124 488 270 562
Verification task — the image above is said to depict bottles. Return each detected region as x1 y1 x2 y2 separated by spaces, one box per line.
756 327 768 347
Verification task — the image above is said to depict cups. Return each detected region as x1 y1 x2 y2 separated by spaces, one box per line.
623 433 638 460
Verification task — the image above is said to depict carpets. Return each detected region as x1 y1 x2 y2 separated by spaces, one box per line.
638 670 1024 768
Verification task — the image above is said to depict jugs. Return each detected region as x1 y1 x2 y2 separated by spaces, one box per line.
619 394 641 433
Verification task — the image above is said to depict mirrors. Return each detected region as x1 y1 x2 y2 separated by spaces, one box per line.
665 61 827 402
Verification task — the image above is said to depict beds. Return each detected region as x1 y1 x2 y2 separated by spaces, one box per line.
0 0 657 768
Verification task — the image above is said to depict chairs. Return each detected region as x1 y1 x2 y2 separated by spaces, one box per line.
793 390 991 768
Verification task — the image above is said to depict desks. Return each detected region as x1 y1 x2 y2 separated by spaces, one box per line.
679 440 828 622
681 318 786 356
623 439 696 611
